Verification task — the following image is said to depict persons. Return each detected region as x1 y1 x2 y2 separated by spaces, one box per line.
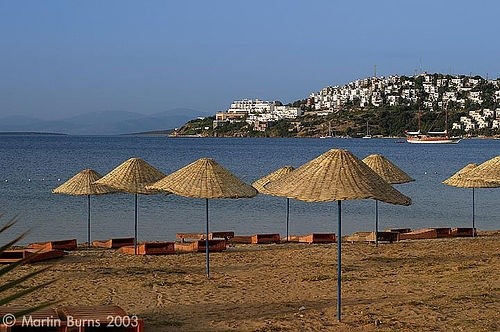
28 178 31 182
4 178 8 184
434 171 437 174
424 171 428 175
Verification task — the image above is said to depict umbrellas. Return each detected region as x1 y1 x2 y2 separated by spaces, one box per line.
441 163 500 237
361 153 416 247
251 166 296 243
461 154 500 186
262 148 411 323
52 168 118 247
91 158 169 255
145 157 257 278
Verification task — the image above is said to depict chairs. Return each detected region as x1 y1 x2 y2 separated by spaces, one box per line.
0 247 70 263
91 237 135 249
120 241 178 255
56 303 146 332
0 305 68 332
234 233 281 244
24 238 78 250
175 231 235 244
399 225 453 239
174 239 227 253
385 228 413 235
281 232 336 244
450 227 477 238
341 231 400 245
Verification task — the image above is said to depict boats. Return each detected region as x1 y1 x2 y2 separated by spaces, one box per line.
407 134 463 144
